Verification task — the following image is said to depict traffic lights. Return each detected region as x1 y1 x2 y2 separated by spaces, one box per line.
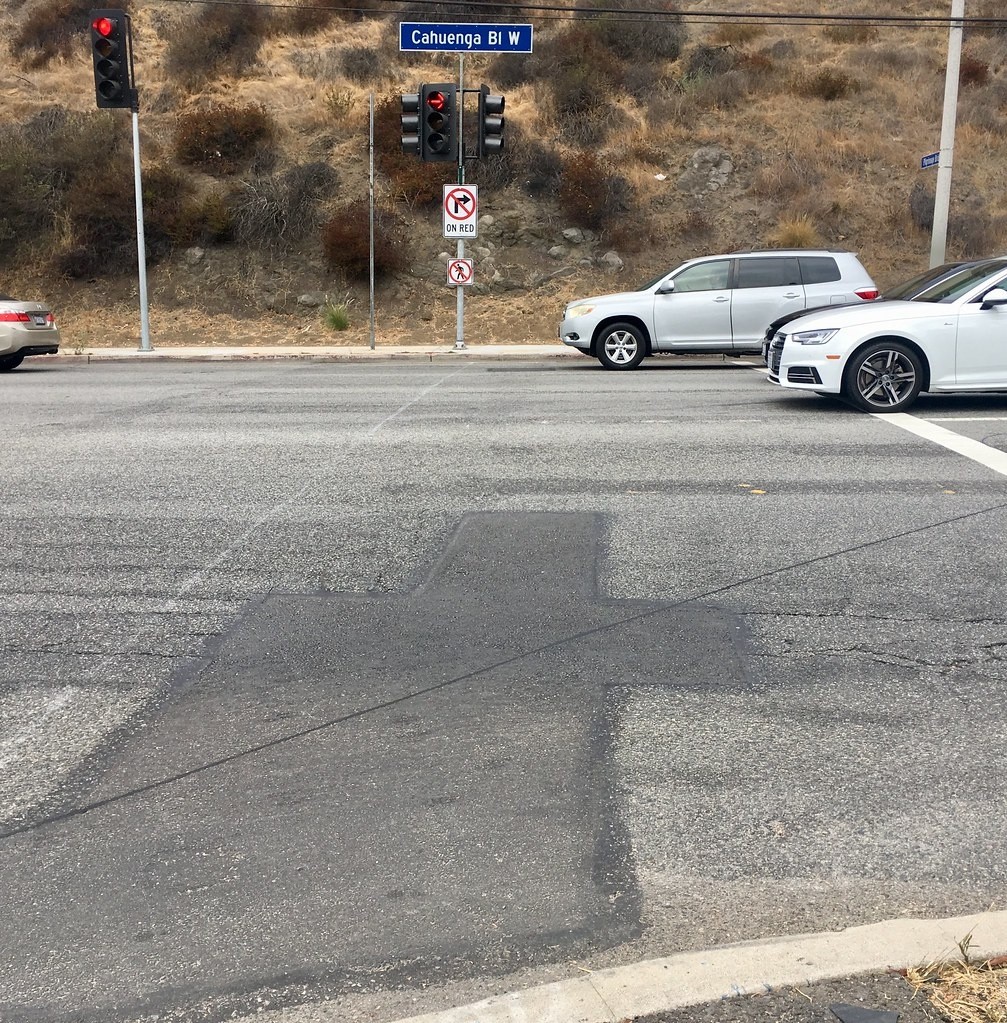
401 83 423 161
480 83 506 156
90 9 130 108
422 83 458 161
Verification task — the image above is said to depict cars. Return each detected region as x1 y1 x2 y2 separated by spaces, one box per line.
762 255 1007 414
0 290 61 373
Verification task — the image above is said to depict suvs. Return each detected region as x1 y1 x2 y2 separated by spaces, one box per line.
558 247 882 372
